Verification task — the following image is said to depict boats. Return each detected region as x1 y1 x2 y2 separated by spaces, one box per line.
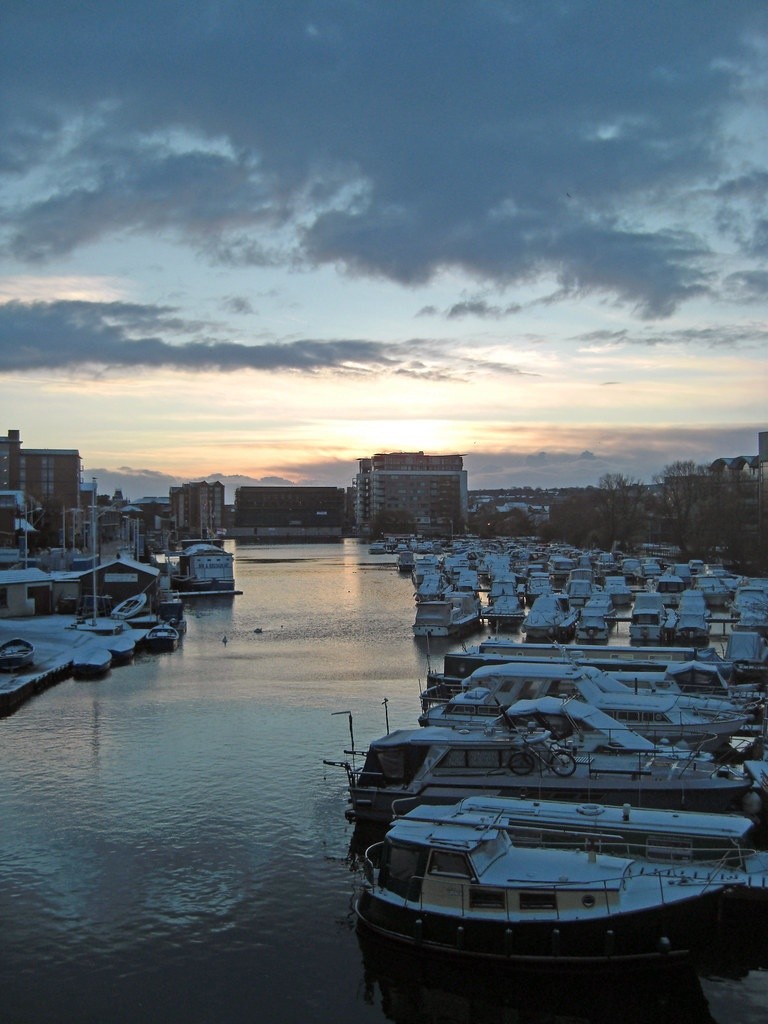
437 638 767 686
323 725 754 827
164 542 244 597
350 795 768 967
0 637 35 672
419 662 755 744
110 593 147 619
368 533 768 660
145 622 179 654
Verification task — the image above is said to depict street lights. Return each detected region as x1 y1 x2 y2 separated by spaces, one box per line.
90 499 130 565
24 501 43 569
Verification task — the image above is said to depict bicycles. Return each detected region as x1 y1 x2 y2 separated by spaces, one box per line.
507 731 578 777
